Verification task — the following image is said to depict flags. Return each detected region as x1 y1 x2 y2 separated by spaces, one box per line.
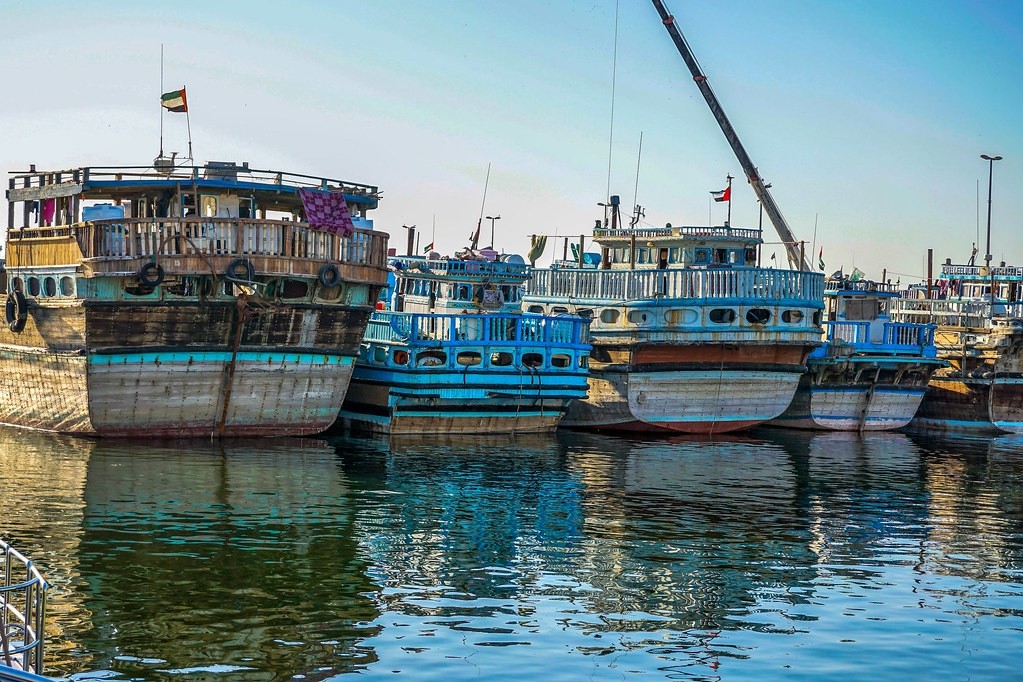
771 253 775 260
424 243 433 254
709 187 729 202
160 88 188 112
818 247 825 271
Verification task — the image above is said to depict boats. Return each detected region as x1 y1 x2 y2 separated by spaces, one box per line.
510 198 827 435
891 154 1023 430
338 215 594 433
769 271 950 437
1 42 391 438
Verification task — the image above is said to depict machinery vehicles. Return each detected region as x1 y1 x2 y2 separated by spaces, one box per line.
651 0 817 272
